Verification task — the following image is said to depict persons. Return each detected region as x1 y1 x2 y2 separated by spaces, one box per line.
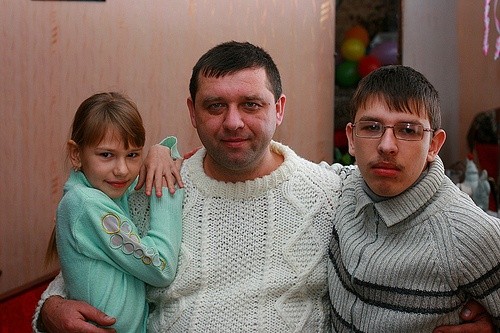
314 65 500 333
33 42 499 333
42 92 184 333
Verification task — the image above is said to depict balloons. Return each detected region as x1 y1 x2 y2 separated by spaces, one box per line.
368 33 398 53
343 40 364 59
357 56 380 77
370 45 400 66
336 62 358 88
344 25 369 45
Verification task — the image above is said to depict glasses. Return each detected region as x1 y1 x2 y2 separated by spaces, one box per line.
354 120 436 141
